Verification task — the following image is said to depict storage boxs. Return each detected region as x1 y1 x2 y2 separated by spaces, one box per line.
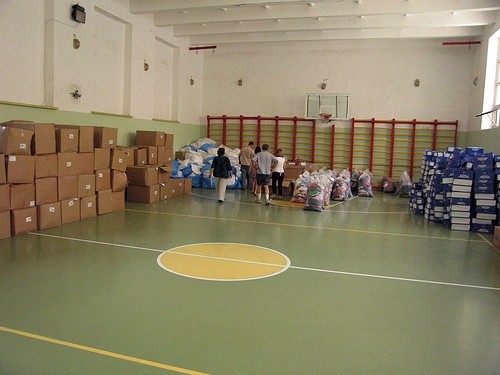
409 147 500 233
0 120 192 240
256 166 325 196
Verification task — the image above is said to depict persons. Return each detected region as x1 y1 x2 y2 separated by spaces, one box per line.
252 144 278 206
270 149 286 200
209 148 232 202
248 146 261 195
238 141 255 190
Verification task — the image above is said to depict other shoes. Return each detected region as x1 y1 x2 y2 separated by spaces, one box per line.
257 199 261 203
266 200 270 206
272 194 277 198
252 192 257 196
279 196 282 199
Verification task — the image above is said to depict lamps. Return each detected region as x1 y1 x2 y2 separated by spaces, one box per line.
72 4 86 23
